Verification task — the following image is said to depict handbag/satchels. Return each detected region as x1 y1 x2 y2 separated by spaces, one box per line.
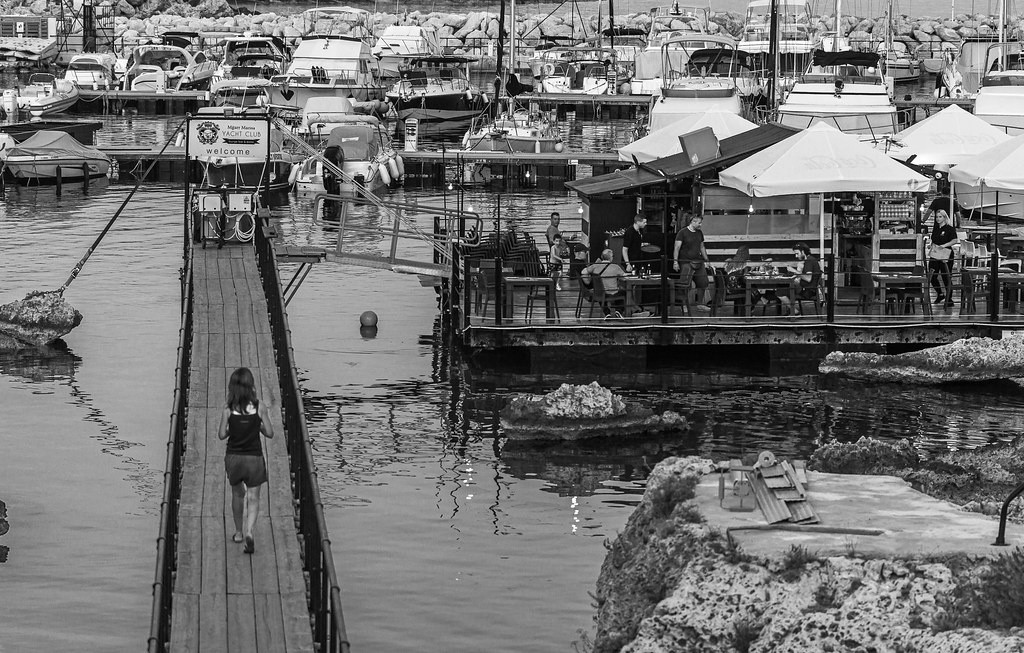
928 244 952 262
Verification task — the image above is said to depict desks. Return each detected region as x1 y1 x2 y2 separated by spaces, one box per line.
502 277 555 324
960 226 1000 248
1003 236 1024 259
744 274 795 322
972 231 1012 252
987 273 1024 318
962 266 1017 313
617 272 676 323
565 241 589 278
871 272 930 320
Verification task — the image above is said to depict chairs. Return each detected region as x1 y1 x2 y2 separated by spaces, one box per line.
458 225 1024 323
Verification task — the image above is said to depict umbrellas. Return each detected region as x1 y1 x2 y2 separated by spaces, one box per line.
618 105 760 165
718 121 931 302
874 103 1024 227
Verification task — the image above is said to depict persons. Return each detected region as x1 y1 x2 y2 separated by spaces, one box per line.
548 233 566 291
929 209 958 311
673 215 712 313
546 212 577 256
218 366 274 555
622 214 650 273
923 186 959 286
783 245 821 319
593 249 629 321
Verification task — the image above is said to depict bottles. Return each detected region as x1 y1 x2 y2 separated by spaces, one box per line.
632 265 636 276
641 267 645 279
647 264 651 275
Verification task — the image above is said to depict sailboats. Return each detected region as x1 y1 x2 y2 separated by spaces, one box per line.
210 0 1024 226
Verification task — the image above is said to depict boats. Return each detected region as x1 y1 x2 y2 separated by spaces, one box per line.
2 130 111 187
122 31 218 91
0 73 79 116
65 53 125 90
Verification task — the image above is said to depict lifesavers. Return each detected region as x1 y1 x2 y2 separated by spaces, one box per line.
544 64 555 76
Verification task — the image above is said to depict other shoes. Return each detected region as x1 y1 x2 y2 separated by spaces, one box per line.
614 311 625 321
944 298 954 308
603 314 611 321
556 284 561 291
785 311 801 320
933 294 945 305
681 306 688 313
696 305 710 312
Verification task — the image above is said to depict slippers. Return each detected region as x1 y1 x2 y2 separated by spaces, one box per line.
243 535 254 554
233 528 243 543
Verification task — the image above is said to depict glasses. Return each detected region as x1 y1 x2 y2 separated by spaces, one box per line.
796 244 801 248
